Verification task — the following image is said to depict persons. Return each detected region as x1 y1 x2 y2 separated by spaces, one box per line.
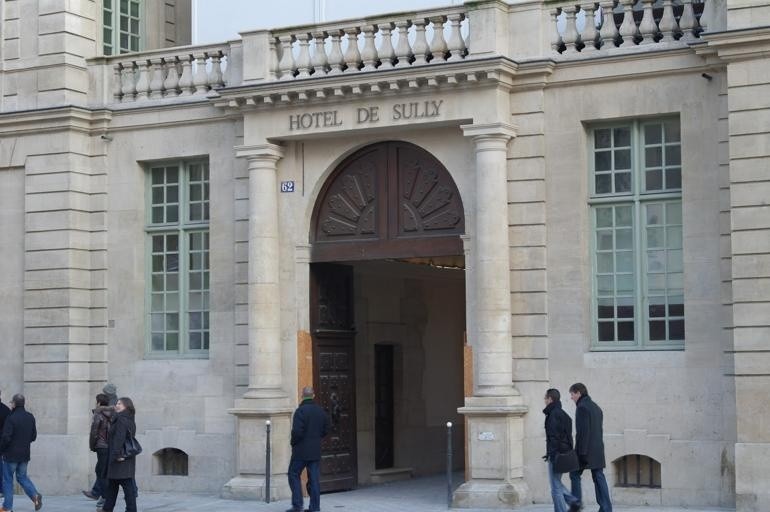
83 383 120 501
0 393 43 511
568 381 613 511
284 385 330 511
90 392 118 508
96 396 142 511
542 388 586 511
0 388 14 498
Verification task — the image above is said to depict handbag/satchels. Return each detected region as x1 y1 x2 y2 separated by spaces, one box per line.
552 448 581 475
114 415 143 463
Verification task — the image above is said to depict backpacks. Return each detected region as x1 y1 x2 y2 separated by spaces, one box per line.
101 411 114 445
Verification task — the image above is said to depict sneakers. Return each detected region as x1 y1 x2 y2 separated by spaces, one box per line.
34 493 42 510
82 489 104 511
0 506 14 512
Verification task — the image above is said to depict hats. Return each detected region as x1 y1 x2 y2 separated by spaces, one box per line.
102 383 117 395
